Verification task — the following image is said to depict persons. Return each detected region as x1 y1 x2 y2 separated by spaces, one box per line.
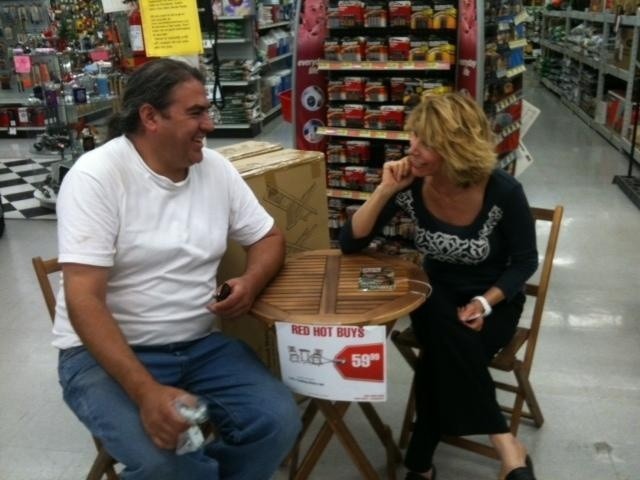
51 58 304 479
340 86 538 479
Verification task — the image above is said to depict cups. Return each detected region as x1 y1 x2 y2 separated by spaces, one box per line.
34 61 127 108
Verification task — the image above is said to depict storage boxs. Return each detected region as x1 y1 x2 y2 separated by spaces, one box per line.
212 140 332 405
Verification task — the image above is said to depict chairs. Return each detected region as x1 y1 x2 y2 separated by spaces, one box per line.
32 255 215 480
391 207 564 479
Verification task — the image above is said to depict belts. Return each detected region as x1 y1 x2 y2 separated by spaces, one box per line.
130 331 215 354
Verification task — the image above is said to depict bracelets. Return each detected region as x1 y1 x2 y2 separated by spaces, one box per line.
471 295 492 317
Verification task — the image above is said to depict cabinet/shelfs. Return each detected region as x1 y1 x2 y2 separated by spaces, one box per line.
196 0 297 131
294 3 535 213
539 2 640 173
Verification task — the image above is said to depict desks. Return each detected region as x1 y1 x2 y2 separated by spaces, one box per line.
249 249 431 480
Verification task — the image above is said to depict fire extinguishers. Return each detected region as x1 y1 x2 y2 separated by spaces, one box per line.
123 0 152 68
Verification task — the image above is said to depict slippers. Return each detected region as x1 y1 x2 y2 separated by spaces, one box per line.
505 454 535 480
403 464 437 480
